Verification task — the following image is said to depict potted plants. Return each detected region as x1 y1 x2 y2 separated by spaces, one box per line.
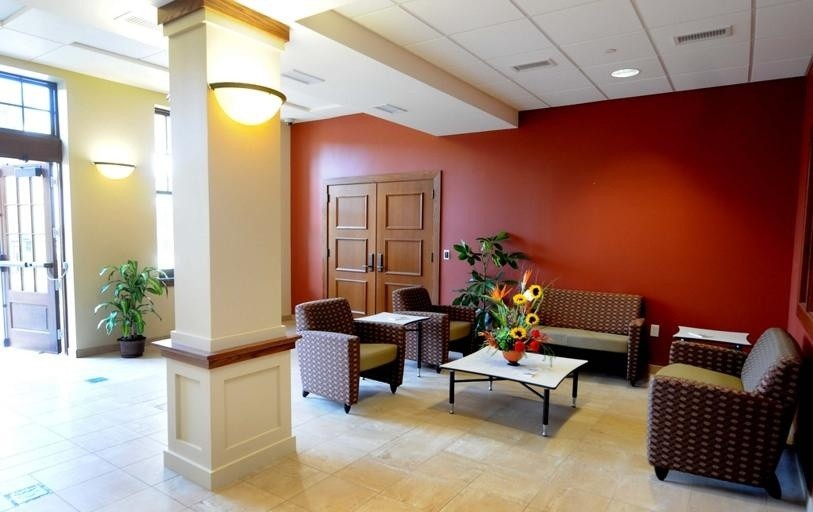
92 259 169 358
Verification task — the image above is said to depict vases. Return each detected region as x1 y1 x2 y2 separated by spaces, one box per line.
501 351 522 367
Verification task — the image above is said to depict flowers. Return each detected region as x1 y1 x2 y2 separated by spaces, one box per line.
477 264 557 367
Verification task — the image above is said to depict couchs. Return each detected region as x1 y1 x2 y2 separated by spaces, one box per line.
292 296 406 415
531 287 645 388
390 285 476 372
645 326 805 499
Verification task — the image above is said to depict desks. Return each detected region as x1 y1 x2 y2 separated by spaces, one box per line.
671 324 753 351
354 311 429 377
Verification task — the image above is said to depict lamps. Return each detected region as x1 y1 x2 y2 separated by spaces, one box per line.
92 160 135 181
209 82 285 126
